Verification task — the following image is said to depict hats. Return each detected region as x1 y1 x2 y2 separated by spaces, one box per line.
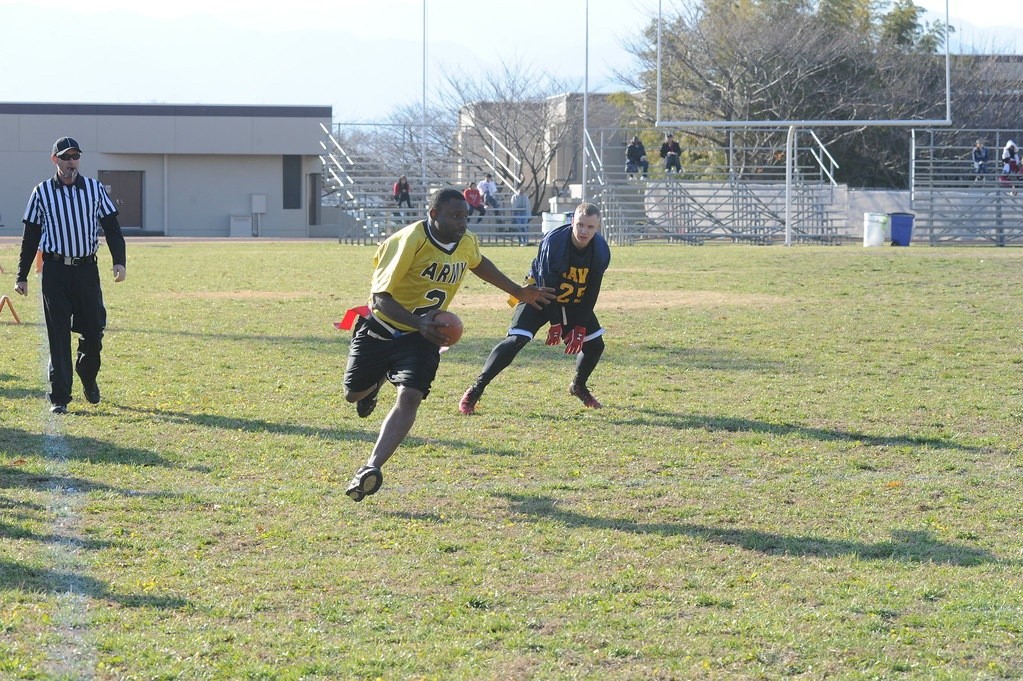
51 137 82 156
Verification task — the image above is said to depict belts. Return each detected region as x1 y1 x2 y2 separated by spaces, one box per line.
46 253 94 267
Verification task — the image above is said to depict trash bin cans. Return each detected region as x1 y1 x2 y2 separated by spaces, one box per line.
886 213 915 246
863 212 888 247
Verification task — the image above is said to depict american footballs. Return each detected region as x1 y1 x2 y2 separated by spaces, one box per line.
432 311 463 346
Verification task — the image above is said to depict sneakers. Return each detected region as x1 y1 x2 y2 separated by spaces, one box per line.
346 466 382 502
459 385 482 414
357 378 384 418
76 369 100 404
569 381 601 408
49 403 66 415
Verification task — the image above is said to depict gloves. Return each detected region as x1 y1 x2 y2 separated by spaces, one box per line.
562 325 587 354
546 325 561 346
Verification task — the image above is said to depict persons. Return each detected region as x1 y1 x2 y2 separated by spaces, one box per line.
510 189 532 246
660 134 683 173
999 140 1022 195
459 203 611 409
972 137 988 182
478 174 501 223
626 135 649 181
14 137 126 410
393 174 414 209
464 181 486 225
341 188 557 503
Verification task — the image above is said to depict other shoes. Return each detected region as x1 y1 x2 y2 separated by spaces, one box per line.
521 243 528 247
665 169 670 173
629 177 633 182
678 169 682 174
640 176 648 181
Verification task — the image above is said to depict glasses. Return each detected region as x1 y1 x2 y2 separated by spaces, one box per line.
56 154 80 160
